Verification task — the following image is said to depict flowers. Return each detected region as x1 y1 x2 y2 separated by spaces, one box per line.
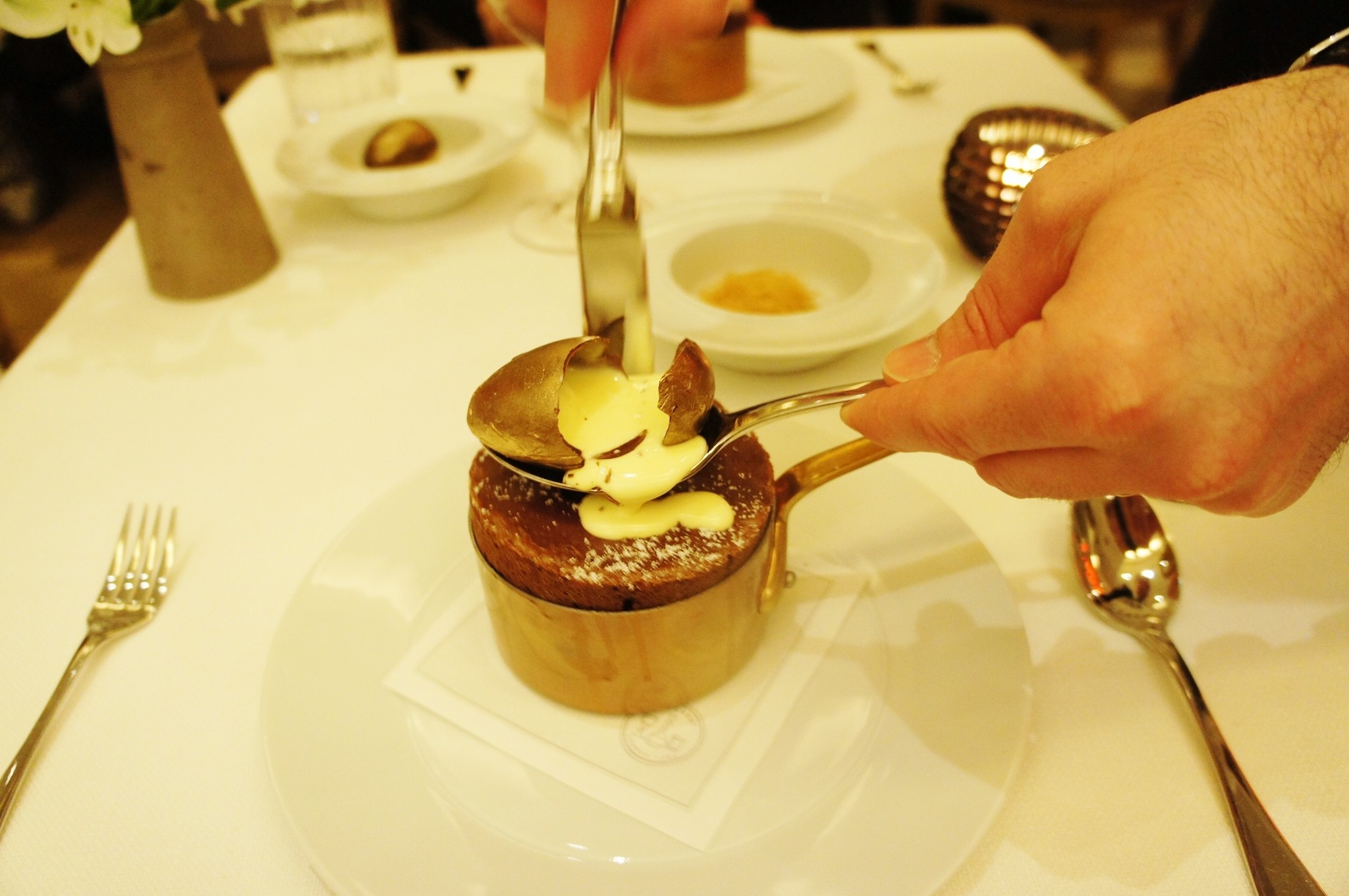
0 0 143 66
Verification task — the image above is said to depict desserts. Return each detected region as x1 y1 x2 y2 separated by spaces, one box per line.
464 333 778 613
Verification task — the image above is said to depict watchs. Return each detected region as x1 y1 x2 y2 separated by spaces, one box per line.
1287 27 1349 72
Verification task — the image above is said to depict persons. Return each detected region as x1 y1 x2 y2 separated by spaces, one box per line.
488 0 1349 517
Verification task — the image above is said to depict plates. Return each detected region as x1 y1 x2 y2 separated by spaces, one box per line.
644 204 949 374
279 98 524 221
615 26 852 142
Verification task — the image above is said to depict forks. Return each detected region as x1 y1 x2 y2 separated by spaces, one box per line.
3 501 177 825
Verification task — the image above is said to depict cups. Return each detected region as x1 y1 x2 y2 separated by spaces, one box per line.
257 4 396 112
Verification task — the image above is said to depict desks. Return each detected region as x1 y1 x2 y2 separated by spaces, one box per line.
0 24 1349 896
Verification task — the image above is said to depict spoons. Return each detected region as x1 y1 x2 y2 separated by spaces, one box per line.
858 37 935 94
1072 495 1329 896
481 373 892 495
